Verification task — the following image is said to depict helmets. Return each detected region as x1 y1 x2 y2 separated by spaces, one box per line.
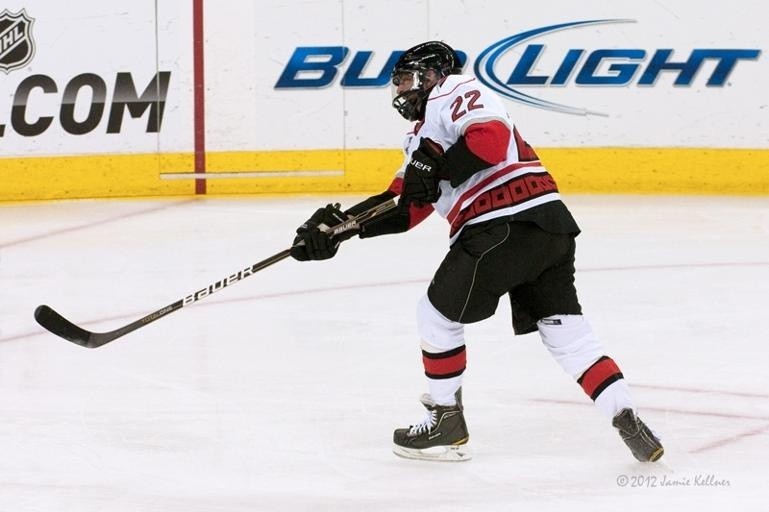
390 40 462 122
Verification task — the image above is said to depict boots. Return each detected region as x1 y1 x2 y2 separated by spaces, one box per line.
612 408 664 463
393 385 469 448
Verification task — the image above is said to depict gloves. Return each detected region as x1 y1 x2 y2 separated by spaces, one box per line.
291 202 348 261
397 136 442 218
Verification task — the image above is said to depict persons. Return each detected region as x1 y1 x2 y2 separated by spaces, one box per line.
290 39 665 464
